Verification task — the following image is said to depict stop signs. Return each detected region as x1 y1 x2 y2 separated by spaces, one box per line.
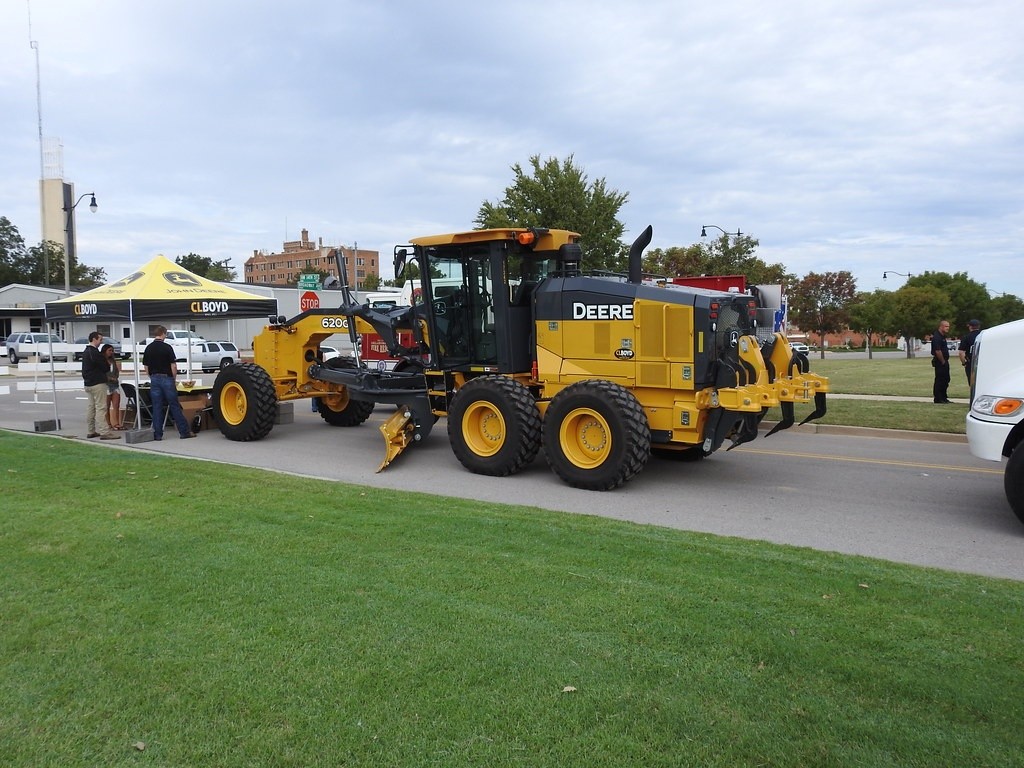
301 290 320 313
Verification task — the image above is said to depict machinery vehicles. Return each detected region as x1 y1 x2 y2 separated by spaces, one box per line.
211 226 829 494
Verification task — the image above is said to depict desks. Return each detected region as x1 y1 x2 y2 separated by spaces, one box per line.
136 385 213 396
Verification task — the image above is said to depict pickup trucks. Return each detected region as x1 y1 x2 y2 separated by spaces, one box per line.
146 329 206 352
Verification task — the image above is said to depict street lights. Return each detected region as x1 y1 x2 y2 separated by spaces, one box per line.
701 225 744 275
61 183 98 347
883 271 914 283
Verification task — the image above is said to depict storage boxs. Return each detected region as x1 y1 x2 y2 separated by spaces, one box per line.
175 395 207 433
199 393 218 430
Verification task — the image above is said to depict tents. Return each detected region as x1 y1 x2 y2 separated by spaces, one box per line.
44 254 279 430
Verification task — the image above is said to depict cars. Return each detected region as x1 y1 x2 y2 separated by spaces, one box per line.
73 337 131 360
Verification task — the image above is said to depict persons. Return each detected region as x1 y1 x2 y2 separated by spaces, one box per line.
958 319 981 386
931 320 954 404
82 332 122 439
100 344 128 430
143 326 197 441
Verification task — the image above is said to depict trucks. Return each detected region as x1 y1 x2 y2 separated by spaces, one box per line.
964 317 1024 526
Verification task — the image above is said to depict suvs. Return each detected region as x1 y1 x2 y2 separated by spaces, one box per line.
788 341 809 356
174 341 241 374
6 332 68 364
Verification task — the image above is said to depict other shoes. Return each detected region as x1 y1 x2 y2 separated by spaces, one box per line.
312 408 317 412
934 398 953 404
87 432 100 438
180 431 196 439
154 433 162 440
100 433 121 440
112 424 127 430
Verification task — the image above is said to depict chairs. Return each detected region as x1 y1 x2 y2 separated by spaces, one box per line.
120 383 152 430
512 261 540 305
151 405 175 431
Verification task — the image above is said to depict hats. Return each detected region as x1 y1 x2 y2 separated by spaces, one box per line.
967 319 980 326
